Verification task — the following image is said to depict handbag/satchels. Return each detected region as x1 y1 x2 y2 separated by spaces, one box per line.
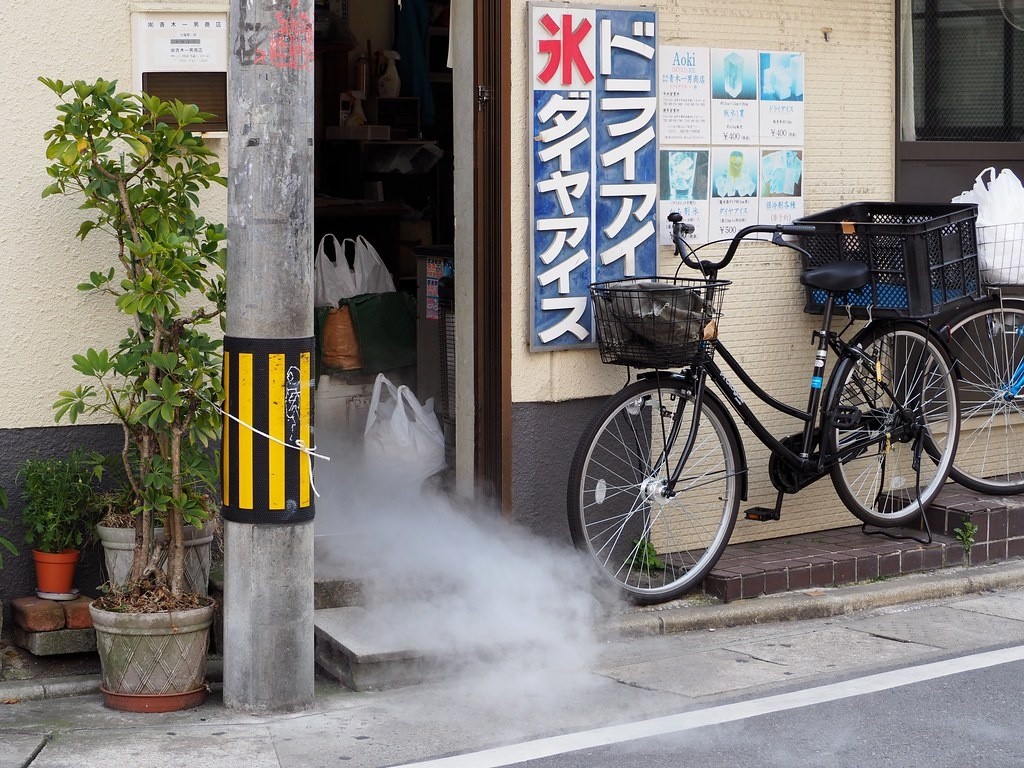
341 235 397 299
949 167 1024 282
314 234 359 309
364 372 449 494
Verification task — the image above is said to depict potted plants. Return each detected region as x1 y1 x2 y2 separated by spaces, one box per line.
18 76 250 713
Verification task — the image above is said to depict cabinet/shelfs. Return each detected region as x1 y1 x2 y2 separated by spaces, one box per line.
314 97 437 281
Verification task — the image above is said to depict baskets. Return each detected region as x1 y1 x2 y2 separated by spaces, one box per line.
586 275 733 370
974 221 1024 287
792 201 984 320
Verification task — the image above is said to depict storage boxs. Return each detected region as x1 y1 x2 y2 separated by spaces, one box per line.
793 201 984 320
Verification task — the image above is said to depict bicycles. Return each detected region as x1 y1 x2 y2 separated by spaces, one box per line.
561 211 963 608
910 162 1024 498
